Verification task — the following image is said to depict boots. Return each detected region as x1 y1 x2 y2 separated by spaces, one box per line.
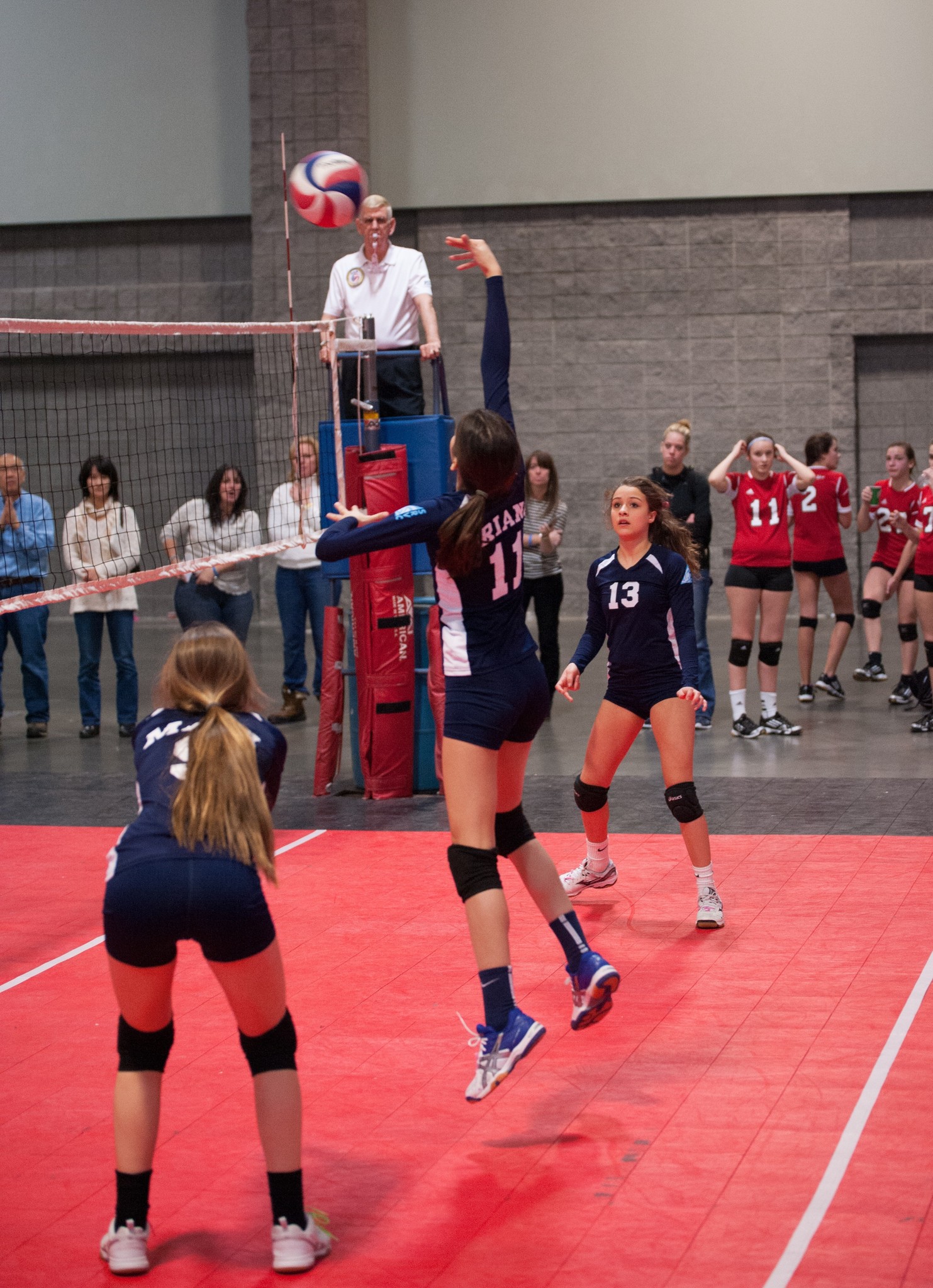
266 688 308 724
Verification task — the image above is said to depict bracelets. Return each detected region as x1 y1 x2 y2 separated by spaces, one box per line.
529 534 534 548
212 566 222 578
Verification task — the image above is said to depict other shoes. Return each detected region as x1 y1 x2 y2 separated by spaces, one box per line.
694 715 713 730
25 721 48 738
79 724 100 738
642 716 652 730
118 722 136 738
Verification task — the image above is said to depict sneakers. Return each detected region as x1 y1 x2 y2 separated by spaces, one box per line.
799 684 817 701
853 661 888 682
559 857 618 897
816 672 845 699
456 1007 547 1102
564 949 621 1031
888 669 919 705
731 713 767 738
910 711 933 732
99 1214 151 1275
694 887 725 929
759 711 803 735
271 1212 333 1272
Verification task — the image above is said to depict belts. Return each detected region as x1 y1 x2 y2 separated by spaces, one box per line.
3 576 32 585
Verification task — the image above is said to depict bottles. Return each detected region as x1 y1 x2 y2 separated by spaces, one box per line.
916 473 930 488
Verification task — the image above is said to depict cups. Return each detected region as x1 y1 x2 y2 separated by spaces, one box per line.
869 486 881 507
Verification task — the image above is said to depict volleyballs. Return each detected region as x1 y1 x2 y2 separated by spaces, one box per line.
289 150 368 229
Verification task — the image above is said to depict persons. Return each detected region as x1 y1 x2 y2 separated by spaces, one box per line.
161 465 263 649
643 418 717 729
266 438 321 724
315 235 620 1102
787 432 853 701
62 455 142 738
708 432 815 739
555 477 725 929
517 450 566 720
318 195 442 420
0 453 55 737
853 441 933 729
98 621 332 1272
